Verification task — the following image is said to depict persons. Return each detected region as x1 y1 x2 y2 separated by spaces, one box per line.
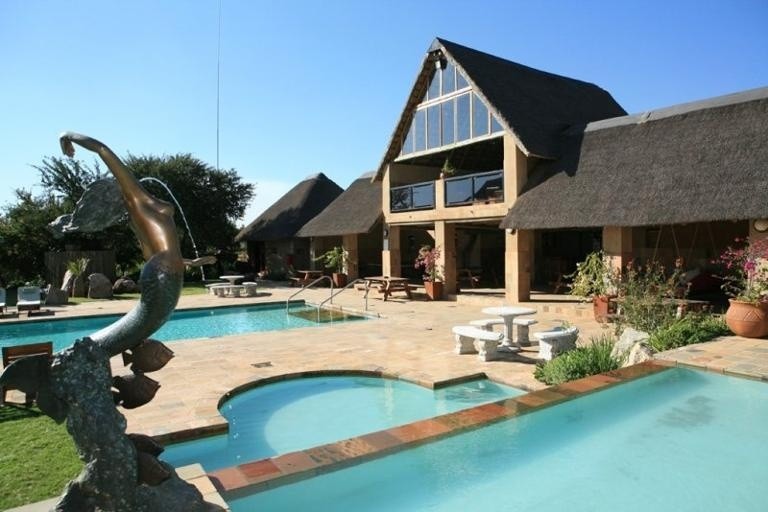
58 129 216 267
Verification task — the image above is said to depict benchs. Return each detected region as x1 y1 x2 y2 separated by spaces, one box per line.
288 276 302 288
378 286 416 300
469 317 537 349
354 283 393 297
535 324 579 363
205 282 230 295
212 285 242 297
242 281 257 297
452 325 502 361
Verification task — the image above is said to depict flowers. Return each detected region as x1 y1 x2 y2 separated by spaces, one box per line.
415 243 442 282
710 232 767 302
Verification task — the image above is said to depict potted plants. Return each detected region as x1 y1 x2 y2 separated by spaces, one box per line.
564 249 619 322
313 243 350 288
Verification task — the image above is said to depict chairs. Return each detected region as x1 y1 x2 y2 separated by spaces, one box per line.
0 287 8 313
1 340 54 406
15 286 41 316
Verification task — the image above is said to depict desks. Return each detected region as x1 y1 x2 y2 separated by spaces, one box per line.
218 276 245 294
363 276 412 301
606 296 710 328
480 306 538 354
287 270 323 289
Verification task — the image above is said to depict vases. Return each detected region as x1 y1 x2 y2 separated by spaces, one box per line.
423 281 442 300
724 298 768 338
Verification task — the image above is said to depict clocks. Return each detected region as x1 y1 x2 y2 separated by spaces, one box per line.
752 219 767 232
